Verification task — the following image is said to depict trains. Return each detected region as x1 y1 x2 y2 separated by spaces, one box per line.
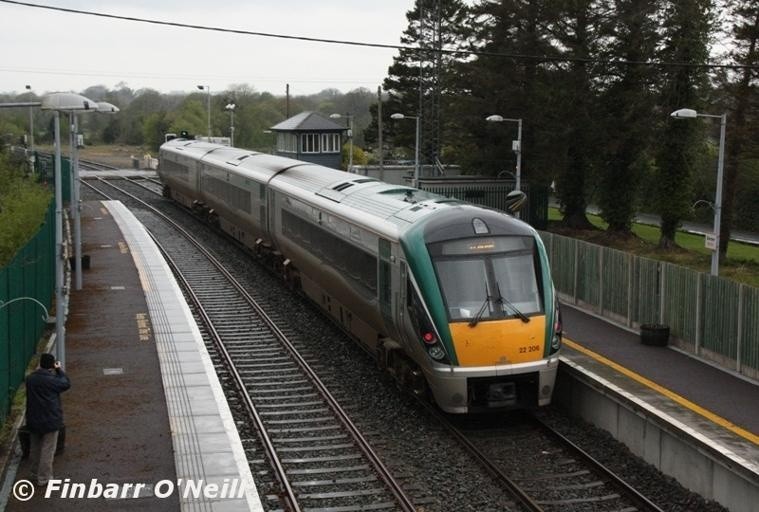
158 136 562 415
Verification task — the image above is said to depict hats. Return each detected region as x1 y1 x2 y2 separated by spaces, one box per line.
40 353 56 369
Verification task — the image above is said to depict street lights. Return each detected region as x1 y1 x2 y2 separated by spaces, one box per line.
390 114 420 190
26 85 34 153
329 113 354 174
484 115 523 220
669 108 727 276
196 84 212 143
39 90 121 376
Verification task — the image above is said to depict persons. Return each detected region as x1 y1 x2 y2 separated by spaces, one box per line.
26 353 71 487
